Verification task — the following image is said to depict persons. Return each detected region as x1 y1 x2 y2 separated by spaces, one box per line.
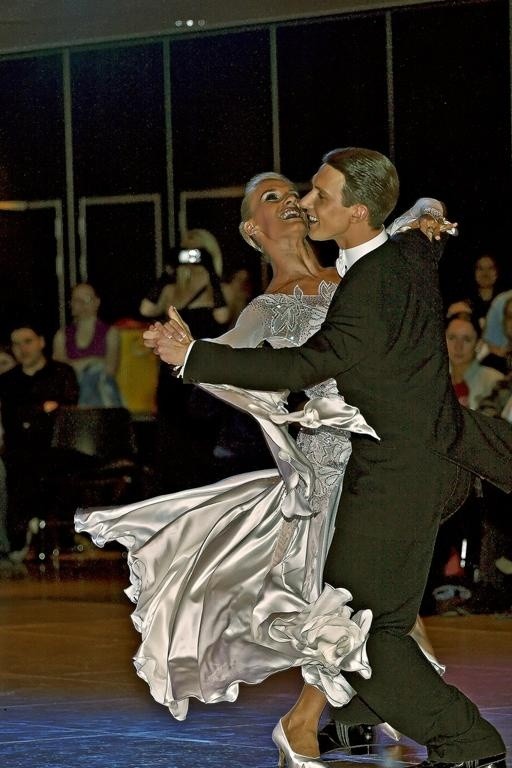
139 164 463 768
140 142 512 768
0 227 510 623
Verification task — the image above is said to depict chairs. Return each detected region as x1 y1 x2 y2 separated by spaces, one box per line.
36 406 142 562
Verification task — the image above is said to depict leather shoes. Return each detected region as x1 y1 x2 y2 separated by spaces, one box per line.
317 721 380 756
403 752 507 768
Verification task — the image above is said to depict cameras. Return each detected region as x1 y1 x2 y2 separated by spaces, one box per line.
177 249 204 265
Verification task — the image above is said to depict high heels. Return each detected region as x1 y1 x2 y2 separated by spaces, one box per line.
271 717 331 768
